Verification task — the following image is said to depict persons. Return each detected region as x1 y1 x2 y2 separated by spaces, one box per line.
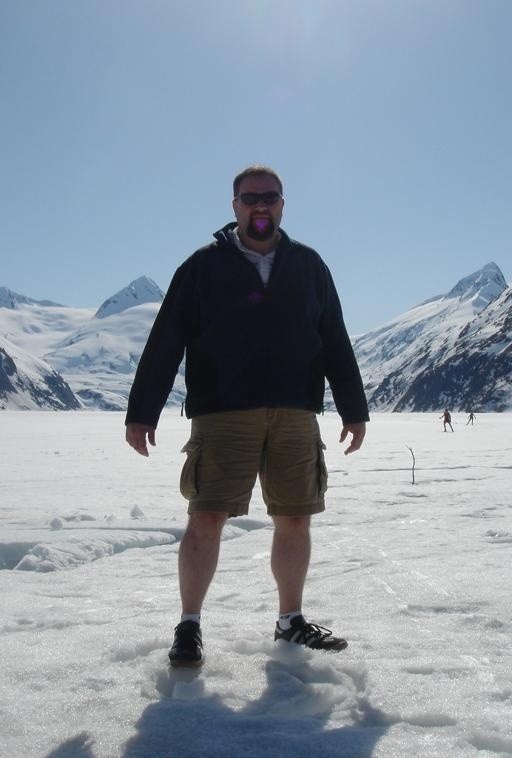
439 408 454 432
466 412 476 425
124 166 369 666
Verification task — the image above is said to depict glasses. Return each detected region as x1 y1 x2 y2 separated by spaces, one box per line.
236 192 282 205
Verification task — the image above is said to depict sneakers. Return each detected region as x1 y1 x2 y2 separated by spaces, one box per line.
168 620 204 667
274 615 348 652
